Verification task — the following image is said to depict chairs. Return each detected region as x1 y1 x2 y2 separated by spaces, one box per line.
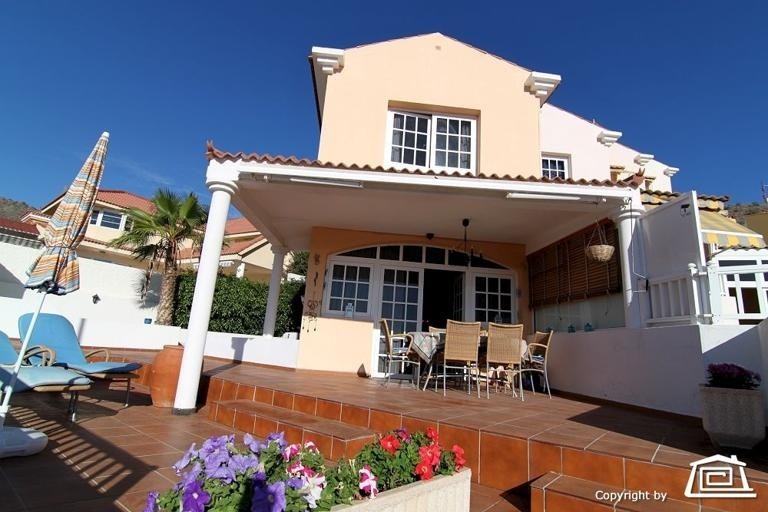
0 330 93 423
379 319 553 402
18 313 142 413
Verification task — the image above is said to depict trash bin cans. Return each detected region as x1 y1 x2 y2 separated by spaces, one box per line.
148 342 185 408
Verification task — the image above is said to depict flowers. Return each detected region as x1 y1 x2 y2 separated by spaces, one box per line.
704 362 761 390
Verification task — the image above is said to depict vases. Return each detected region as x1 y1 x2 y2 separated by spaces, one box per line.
697 382 768 449
149 345 184 407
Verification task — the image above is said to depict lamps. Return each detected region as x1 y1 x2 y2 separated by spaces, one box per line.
447 218 483 267
289 176 365 189
504 192 581 201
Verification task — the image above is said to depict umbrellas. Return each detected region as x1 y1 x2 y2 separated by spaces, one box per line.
0 128 111 428
